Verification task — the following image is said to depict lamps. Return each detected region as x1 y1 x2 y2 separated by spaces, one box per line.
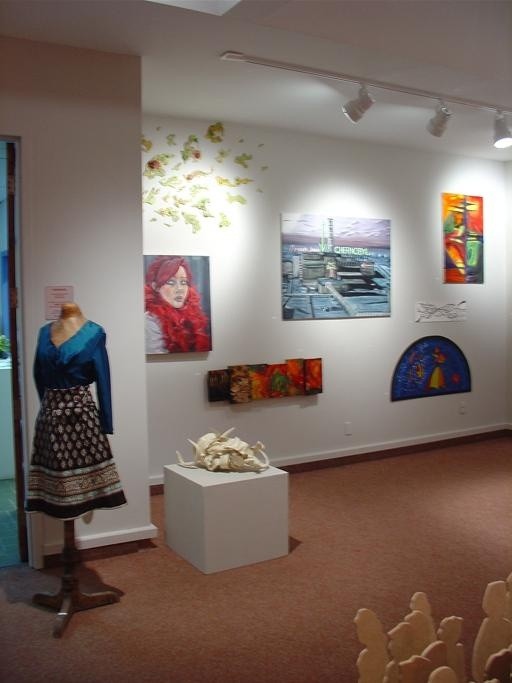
340 82 512 151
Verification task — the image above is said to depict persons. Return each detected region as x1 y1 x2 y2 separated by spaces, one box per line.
143 256 209 354
23 302 128 522
